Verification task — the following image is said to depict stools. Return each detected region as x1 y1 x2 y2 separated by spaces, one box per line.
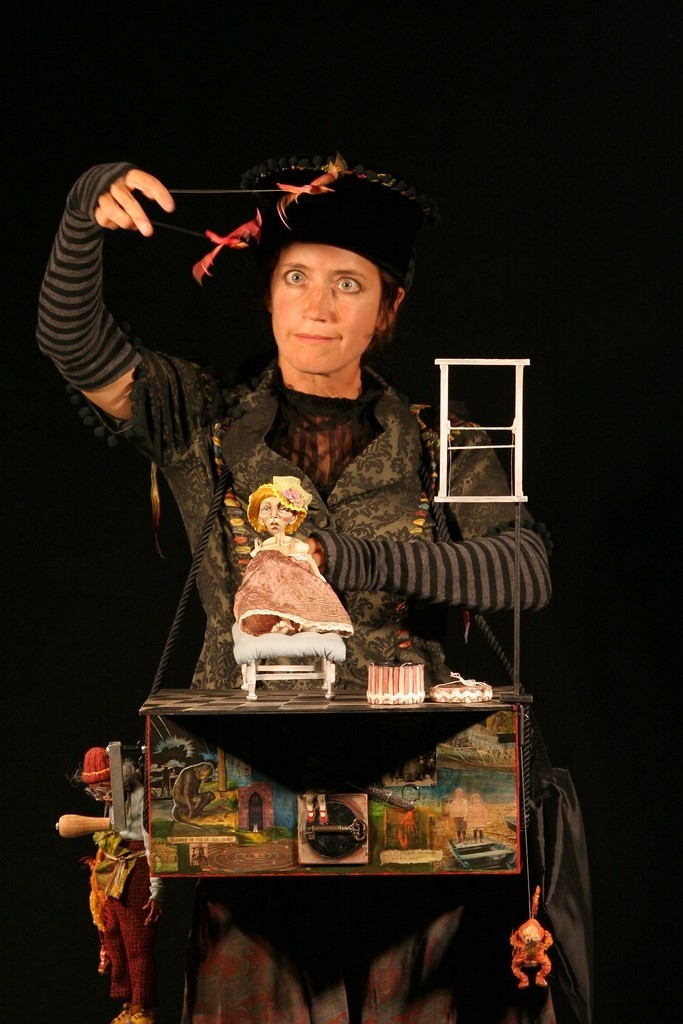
231 623 346 701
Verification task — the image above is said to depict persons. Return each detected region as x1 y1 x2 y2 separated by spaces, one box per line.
34 147 601 1024
79 747 165 1023
223 474 356 637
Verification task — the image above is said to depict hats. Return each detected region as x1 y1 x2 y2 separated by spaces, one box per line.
238 153 442 294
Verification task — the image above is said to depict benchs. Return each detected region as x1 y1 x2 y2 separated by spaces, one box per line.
460 849 513 859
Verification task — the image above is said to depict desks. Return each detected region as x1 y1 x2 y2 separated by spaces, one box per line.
167 824 236 866
